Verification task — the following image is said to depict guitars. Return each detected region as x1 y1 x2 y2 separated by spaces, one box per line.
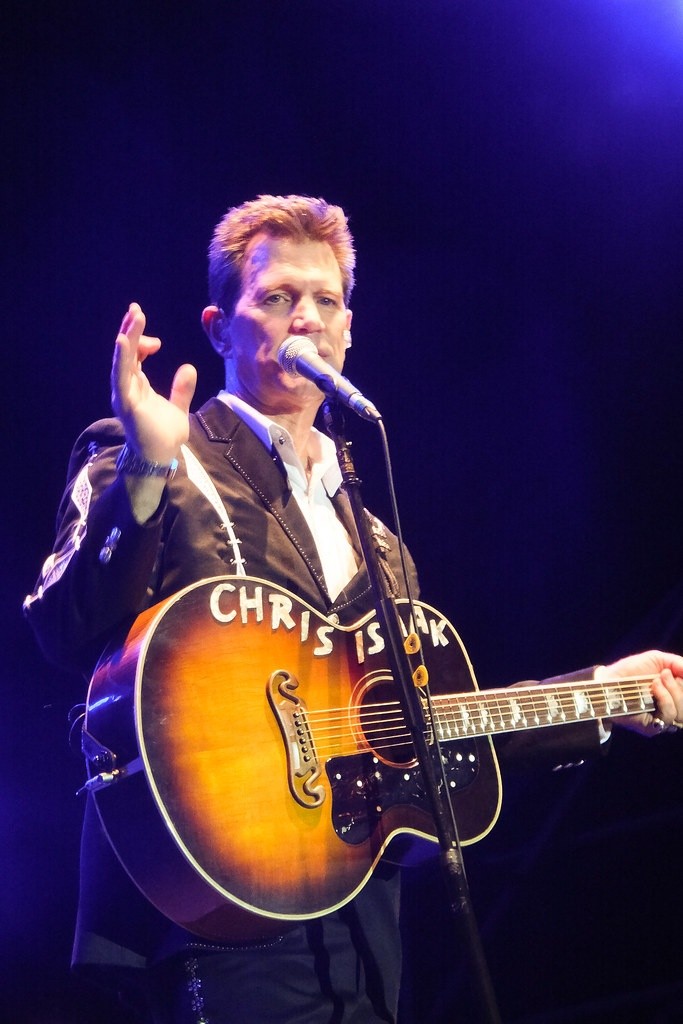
78 571 662 945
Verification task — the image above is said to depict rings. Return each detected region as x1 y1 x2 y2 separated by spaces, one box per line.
651 719 669 733
672 719 683 730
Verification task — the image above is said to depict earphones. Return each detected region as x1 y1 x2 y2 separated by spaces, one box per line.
217 319 227 342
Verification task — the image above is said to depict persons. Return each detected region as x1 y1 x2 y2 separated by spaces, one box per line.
21 193 683 1024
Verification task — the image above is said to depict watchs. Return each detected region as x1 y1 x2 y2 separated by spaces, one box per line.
112 443 180 480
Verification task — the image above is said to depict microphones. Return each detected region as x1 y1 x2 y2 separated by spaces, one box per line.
277 336 382 422
